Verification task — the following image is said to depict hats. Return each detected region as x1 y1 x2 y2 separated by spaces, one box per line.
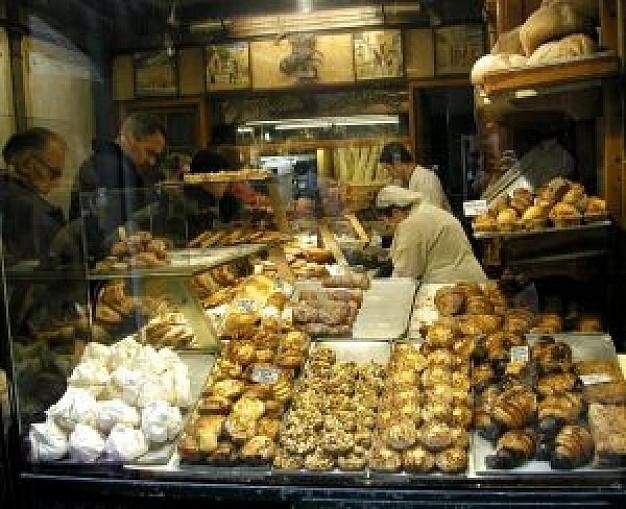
374 184 426 211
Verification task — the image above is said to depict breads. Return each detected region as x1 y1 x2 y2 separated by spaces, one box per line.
92 228 173 273
471 331 626 471
368 335 473 475
176 331 311 465
418 277 604 335
219 269 372 340
271 345 387 472
469 0 598 107
22 335 192 467
470 173 607 234
186 223 293 247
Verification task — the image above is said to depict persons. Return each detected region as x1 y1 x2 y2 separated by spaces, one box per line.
178 149 237 240
380 141 455 215
70 111 167 256
0 127 68 382
373 186 491 283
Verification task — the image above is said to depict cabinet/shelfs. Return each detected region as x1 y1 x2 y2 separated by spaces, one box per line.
1 182 288 420
468 52 622 269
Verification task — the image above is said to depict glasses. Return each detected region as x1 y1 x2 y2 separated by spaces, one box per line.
33 158 64 184
